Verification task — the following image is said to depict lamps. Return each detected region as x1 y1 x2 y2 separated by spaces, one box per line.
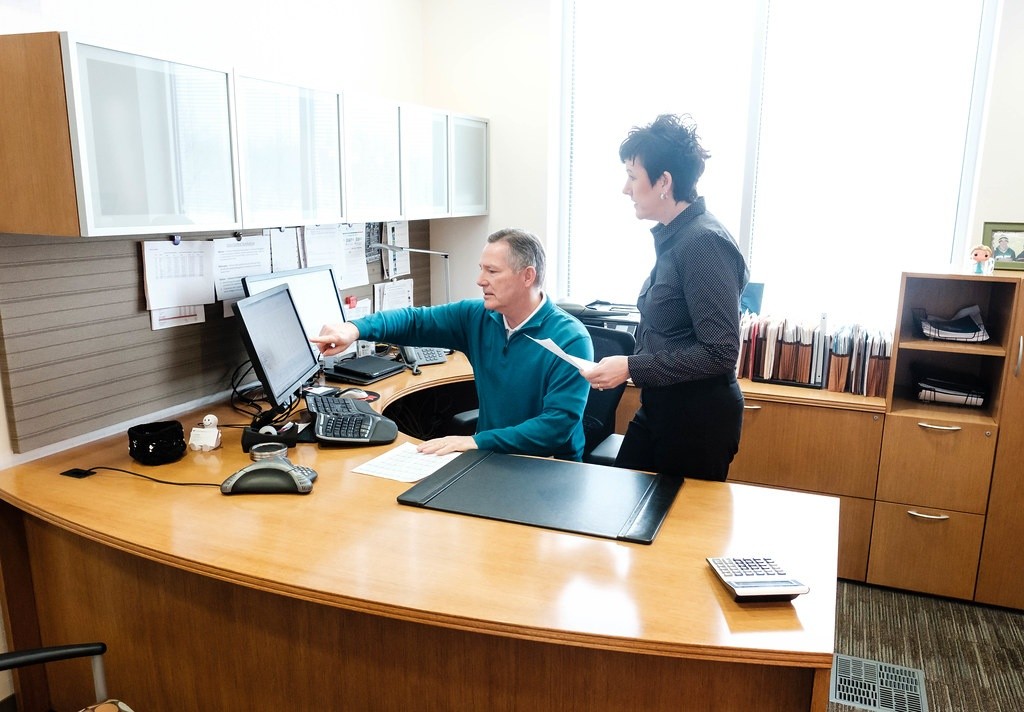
368 243 451 304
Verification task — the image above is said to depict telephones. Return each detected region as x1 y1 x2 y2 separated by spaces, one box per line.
397 345 447 367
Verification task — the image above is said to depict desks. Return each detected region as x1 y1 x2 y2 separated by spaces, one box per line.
0 353 839 712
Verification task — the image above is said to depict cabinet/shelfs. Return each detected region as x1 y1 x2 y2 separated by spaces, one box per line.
2 20 489 236
866 269 1024 611
616 383 887 582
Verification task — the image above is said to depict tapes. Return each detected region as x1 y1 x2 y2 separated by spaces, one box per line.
259 426 277 435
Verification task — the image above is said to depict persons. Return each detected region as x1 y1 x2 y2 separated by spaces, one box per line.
308 229 593 462
970 246 992 274
994 234 1024 262
578 115 751 482
190 414 221 451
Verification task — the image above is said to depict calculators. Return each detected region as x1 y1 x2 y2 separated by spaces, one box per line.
706 558 811 602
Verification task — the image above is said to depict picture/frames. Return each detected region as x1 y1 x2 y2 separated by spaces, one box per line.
984 221 1024 272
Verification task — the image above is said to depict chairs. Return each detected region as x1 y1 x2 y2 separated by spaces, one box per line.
452 323 635 463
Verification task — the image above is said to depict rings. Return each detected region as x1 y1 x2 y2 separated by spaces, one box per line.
598 382 603 391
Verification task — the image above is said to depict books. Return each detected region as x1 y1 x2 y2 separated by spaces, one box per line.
737 313 893 398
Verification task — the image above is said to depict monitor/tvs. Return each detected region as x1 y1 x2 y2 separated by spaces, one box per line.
232 264 357 443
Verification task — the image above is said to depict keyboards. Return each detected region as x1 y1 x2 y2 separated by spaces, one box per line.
304 394 398 447
